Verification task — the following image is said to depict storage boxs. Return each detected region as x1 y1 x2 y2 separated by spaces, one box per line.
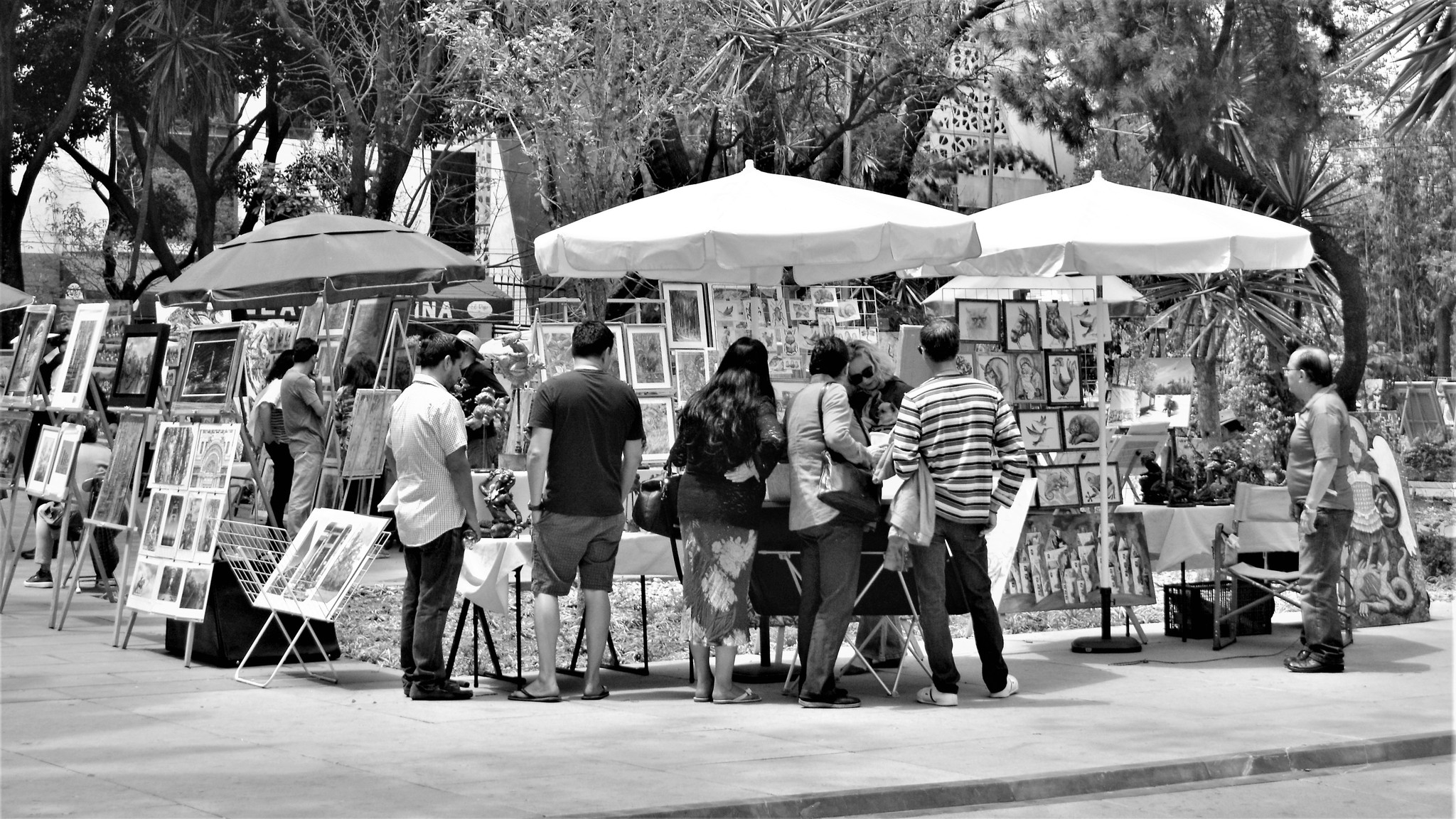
1164 580 1232 641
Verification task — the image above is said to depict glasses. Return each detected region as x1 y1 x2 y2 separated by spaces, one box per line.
848 366 873 385
1282 367 1309 376
917 345 928 354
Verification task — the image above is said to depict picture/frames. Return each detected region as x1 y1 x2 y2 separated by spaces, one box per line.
0 411 86 504
251 295 411 521
537 280 718 461
109 324 170 407
51 303 109 407
170 324 246 416
952 298 1123 507
0 305 55 408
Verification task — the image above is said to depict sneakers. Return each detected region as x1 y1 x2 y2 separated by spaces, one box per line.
990 673 1019 698
915 683 957 706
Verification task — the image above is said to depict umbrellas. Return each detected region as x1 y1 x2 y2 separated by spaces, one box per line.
896 169 1314 653
156 211 485 511
0 282 37 312
534 159 980 341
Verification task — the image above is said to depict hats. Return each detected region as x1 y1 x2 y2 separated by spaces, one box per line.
450 330 485 361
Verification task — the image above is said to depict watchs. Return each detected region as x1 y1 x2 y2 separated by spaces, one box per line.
528 498 544 511
1302 504 1317 513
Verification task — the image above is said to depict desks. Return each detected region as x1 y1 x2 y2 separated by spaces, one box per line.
378 471 549 551
456 531 685 685
1113 503 1234 642
751 507 970 699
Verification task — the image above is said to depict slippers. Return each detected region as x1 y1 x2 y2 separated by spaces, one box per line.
693 697 713 701
582 686 610 700
712 688 764 703
507 687 561 703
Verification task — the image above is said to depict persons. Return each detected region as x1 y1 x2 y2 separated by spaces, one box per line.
385 332 481 700
1137 446 1287 508
478 467 523 538
246 349 295 553
1281 344 1355 673
333 352 390 559
281 337 329 541
506 319 645 702
787 319 1028 709
450 330 510 468
668 336 783 703
21 328 116 559
24 414 124 588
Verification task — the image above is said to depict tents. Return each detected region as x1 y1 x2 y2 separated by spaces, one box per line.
395 275 514 323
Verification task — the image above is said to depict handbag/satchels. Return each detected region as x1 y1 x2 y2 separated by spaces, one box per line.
632 458 682 539
816 447 881 523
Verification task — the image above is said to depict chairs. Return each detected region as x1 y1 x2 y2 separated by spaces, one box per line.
60 476 119 593
1213 481 1353 651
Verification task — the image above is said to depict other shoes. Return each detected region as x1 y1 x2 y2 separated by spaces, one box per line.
871 659 900 669
23 569 54 588
21 545 57 559
798 689 861 708
1288 657 1345 672
1283 650 1311 667
841 658 873 676
99 576 116 585
405 680 475 700
